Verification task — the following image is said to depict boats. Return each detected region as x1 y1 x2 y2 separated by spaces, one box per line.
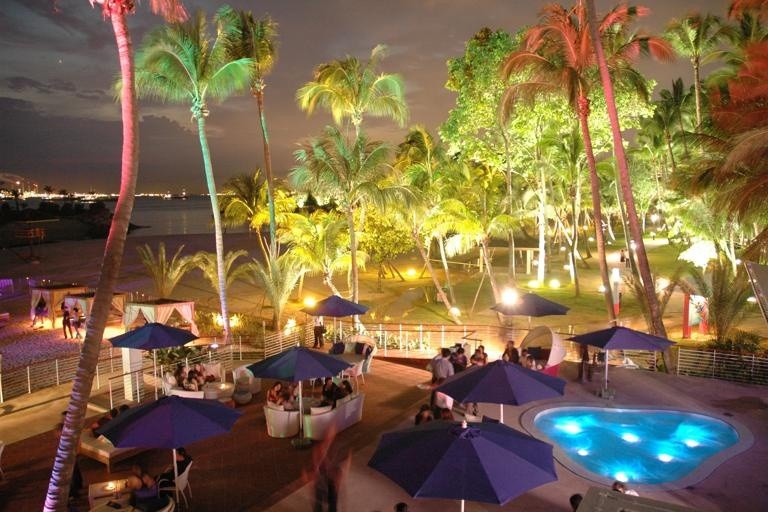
164 194 189 200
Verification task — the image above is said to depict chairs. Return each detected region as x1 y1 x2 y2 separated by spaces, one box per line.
263 335 378 442
163 361 262 411
148 447 200 511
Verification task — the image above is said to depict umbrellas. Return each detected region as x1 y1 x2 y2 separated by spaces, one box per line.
300 294 370 343
490 290 571 330
563 324 679 391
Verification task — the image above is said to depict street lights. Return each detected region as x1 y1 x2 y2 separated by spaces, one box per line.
564 228 570 265
612 267 621 317
651 213 657 239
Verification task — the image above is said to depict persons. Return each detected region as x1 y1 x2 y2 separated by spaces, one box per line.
429 340 489 416
613 480 639 496
394 502 408 512
175 364 205 392
90 405 129 438
414 403 456 426
267 382 299 412
120 447 193 512
502 341 545 372
31 298 46 328
313 316 324 348
56 411 83 499
618 249 627 264
71 308 83 340
312 377 354 410
617 350 634 366
60 306 73 340
576 342 592 383
569 493 583 512
